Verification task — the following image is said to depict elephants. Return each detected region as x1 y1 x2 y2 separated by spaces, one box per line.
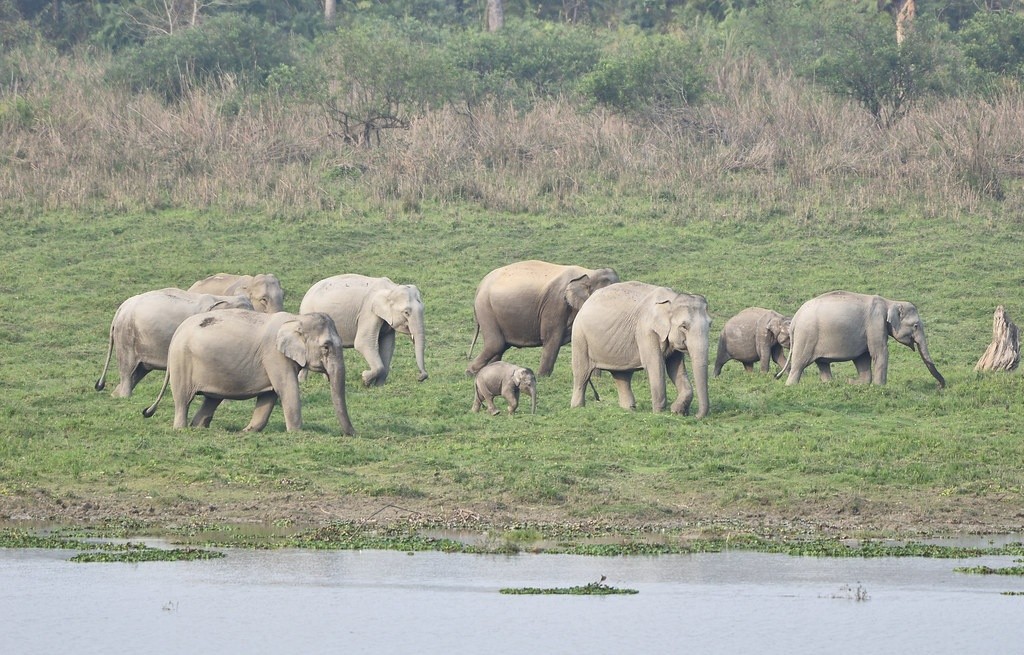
298 274 428 388
774 290 946 389
465 260 621 378
713 307 792 378
185 272 285 315
570 280 713 420
142 308 356 437
471 361 536 416
95 287 255 399
